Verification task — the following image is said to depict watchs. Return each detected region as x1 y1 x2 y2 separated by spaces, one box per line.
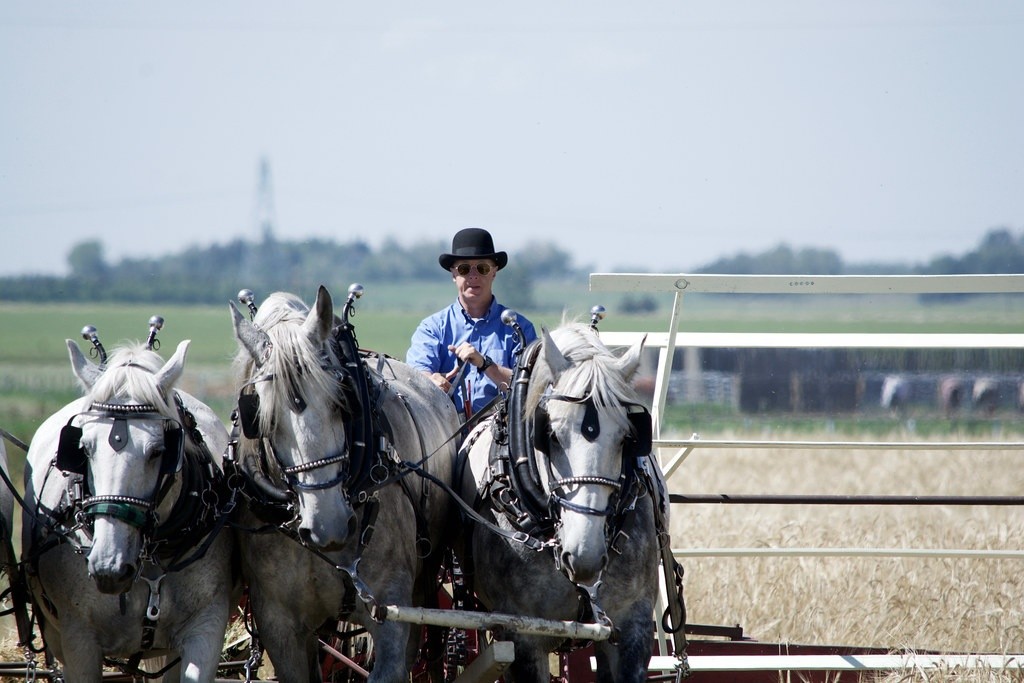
477 355 492 375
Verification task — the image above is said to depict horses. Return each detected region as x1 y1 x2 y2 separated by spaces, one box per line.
0 283 671 683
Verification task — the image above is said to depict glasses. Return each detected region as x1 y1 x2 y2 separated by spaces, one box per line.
452 263 496 277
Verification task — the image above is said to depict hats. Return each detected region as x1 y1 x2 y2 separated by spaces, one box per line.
439 228 507 272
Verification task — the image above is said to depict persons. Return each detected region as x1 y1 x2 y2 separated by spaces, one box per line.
404 228 536 425
